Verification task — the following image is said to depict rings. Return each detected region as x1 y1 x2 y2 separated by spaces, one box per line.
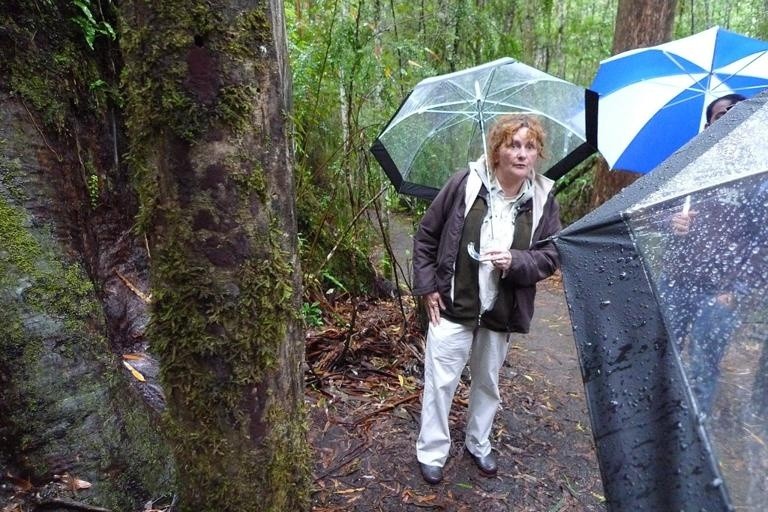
431 301 438 307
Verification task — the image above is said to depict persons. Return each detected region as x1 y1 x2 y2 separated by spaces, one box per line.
645 90 767 418
414 112 562 484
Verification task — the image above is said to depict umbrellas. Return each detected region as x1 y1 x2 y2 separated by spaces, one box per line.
371 55 603 267
587 24 767 225
548 86 767 512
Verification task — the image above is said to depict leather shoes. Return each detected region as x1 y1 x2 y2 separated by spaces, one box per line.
475 453 498 477
419 463 443 484
